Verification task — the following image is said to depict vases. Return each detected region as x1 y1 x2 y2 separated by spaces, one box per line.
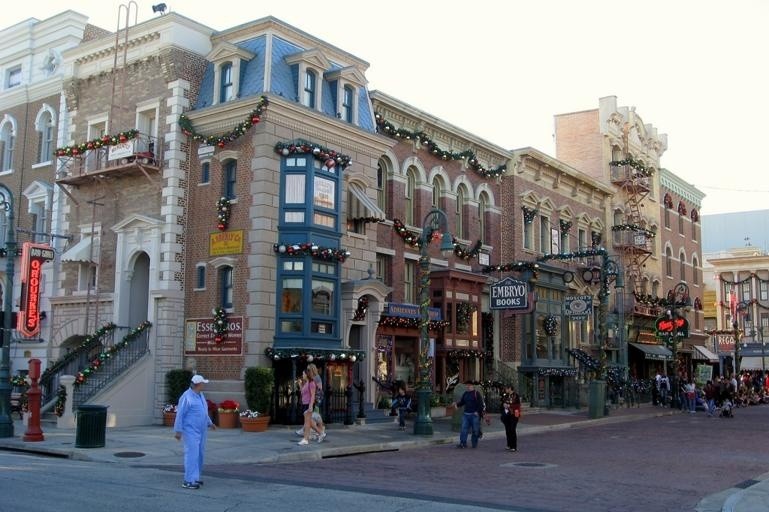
163 412 178 426
209 413 238 428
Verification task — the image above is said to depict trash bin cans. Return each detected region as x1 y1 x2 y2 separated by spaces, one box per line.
74 405 111 448
587 381 607 418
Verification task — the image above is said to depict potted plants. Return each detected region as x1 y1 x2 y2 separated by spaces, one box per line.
238 366 276 430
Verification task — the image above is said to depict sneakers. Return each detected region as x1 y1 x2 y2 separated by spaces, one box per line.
505 446 516 452
196 481 203 485
182 481 200 489
295 429 326 445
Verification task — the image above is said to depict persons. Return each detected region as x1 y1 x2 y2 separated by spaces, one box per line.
448 380 485 450
297 368 327 447
172 374 219 490
389 384 413 432
498 380 522 454
649 368 768 418
294 364 329 441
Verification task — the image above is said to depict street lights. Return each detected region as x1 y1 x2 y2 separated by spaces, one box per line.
669 280 693 410
413 207 458 437
588 257 624 419
733 302 754 404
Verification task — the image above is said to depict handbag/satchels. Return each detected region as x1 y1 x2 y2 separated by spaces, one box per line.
315 389 324 404
480 403 486 417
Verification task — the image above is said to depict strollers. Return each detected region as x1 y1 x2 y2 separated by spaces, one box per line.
717 396 734 418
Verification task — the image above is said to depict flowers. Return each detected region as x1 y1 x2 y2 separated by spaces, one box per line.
163 403 179 413
206 400 238 412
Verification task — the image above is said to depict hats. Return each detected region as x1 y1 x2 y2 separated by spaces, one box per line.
191 376 209 384
